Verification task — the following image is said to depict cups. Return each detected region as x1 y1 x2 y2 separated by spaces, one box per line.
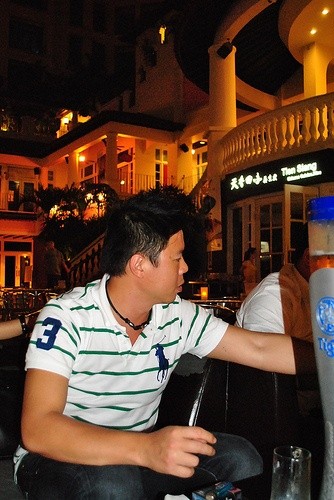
271 445 312 500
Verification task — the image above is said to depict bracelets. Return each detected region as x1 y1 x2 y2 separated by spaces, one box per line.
17 316 30 334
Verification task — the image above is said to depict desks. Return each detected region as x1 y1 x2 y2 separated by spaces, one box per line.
9 288 51 290
188 299 242 302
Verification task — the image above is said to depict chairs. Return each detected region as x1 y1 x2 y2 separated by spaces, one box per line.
0 287 300 455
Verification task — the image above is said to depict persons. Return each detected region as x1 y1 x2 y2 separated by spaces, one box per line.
232 221 314 423
41 239 71 293
12 199 317 500
0 312 41 340
241 248 257 283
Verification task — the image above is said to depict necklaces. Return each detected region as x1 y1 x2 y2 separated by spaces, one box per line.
106 276 153 331
